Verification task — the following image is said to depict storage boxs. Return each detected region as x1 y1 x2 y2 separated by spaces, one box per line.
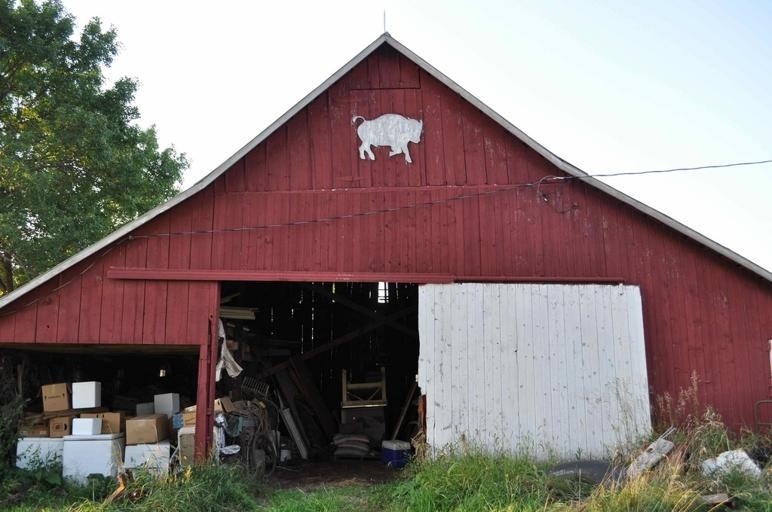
12 379 235 493
